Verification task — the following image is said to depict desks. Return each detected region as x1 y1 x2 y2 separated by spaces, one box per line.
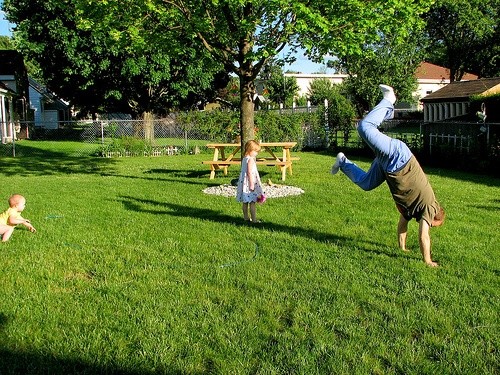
206 142 297 181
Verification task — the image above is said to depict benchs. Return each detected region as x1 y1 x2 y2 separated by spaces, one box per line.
218 157 300 160
202 162 292 165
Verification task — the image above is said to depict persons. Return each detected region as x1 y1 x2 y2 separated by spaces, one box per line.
332 85 445 267
237 140 264 224
0 195 36 243
